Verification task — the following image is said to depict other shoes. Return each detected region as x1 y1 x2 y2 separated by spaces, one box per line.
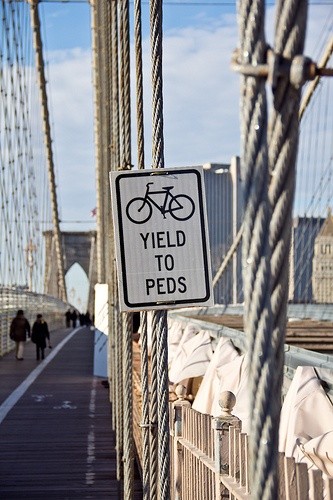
16 355 24 361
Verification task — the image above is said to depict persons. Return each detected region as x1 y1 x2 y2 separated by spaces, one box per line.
65 308 95 329
30 314 52 360
10 309 31 361
